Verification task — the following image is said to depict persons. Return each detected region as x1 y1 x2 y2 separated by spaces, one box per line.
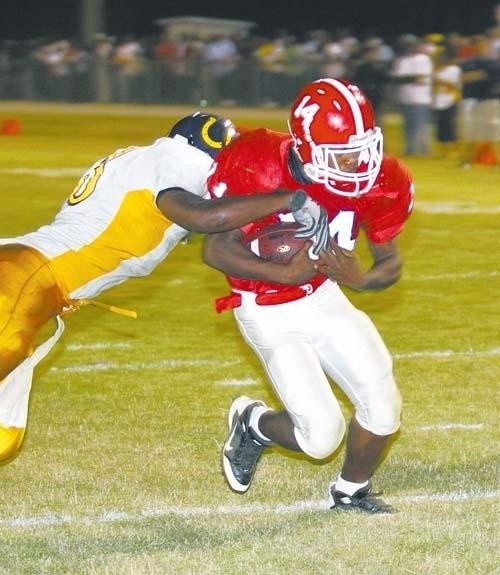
387 31 464 157
0 27 500 113
0 113 329 468
203 79 413 514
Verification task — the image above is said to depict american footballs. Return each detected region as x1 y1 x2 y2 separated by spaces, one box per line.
242 222 311 265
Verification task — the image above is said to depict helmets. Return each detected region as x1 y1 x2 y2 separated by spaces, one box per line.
287 78 375 164
168 111 236 159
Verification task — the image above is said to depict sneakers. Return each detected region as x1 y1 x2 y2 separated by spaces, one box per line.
329 480 394 513
223 396 275 494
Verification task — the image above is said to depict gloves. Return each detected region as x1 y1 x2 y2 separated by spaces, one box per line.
291 190 330 261
